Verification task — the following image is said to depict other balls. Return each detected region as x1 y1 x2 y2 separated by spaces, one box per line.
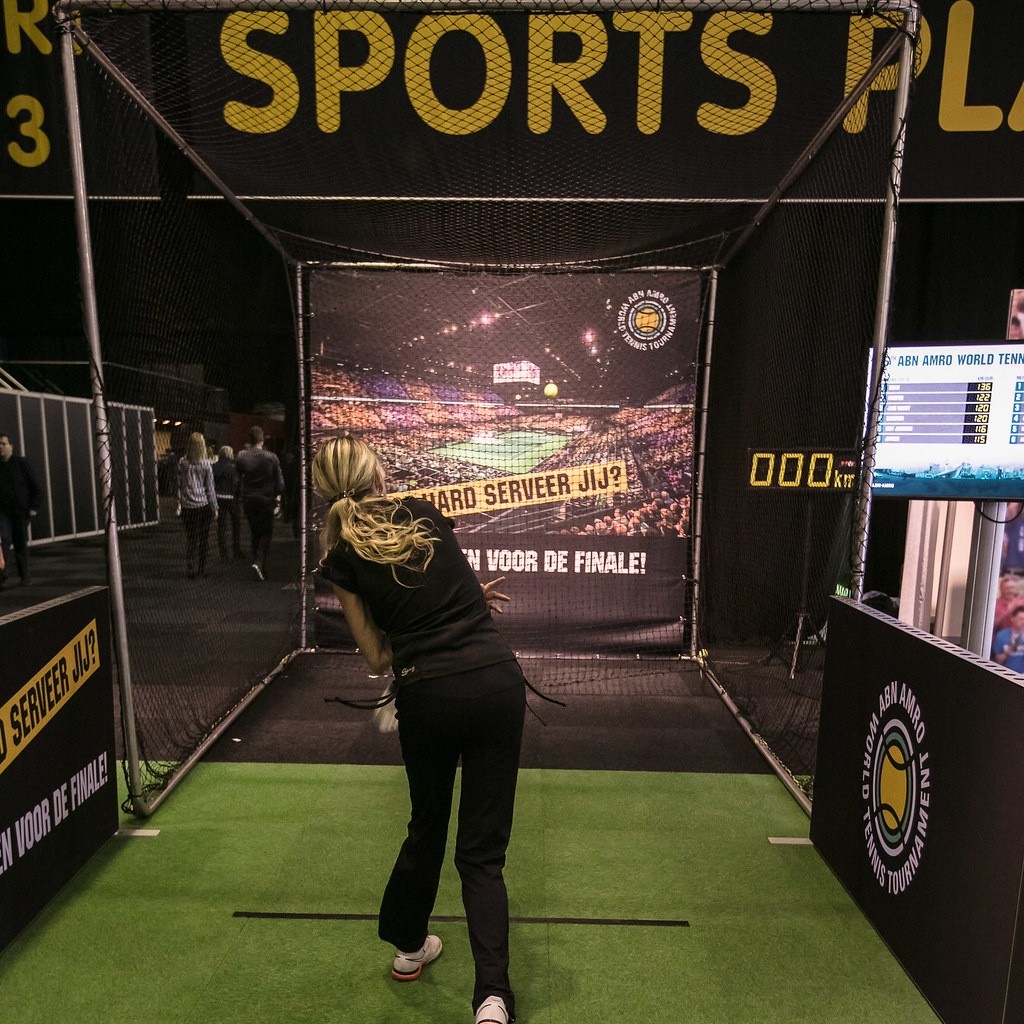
544 383 559 397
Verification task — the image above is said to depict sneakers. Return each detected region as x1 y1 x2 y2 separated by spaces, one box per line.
390 934 443 981
475 995 509 1024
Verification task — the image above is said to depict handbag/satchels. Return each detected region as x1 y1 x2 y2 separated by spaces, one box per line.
371 679 398 734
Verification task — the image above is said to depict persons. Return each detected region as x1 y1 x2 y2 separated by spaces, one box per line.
310 437 526 1024
309 356 696 539
0 434 43 584
162 425 285 581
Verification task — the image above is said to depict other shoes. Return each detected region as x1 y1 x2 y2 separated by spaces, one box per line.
20 571 32 586
198 557 206 574
251 558 267 582
232 552 247 561
185 560 196 579
0 572 9 583
